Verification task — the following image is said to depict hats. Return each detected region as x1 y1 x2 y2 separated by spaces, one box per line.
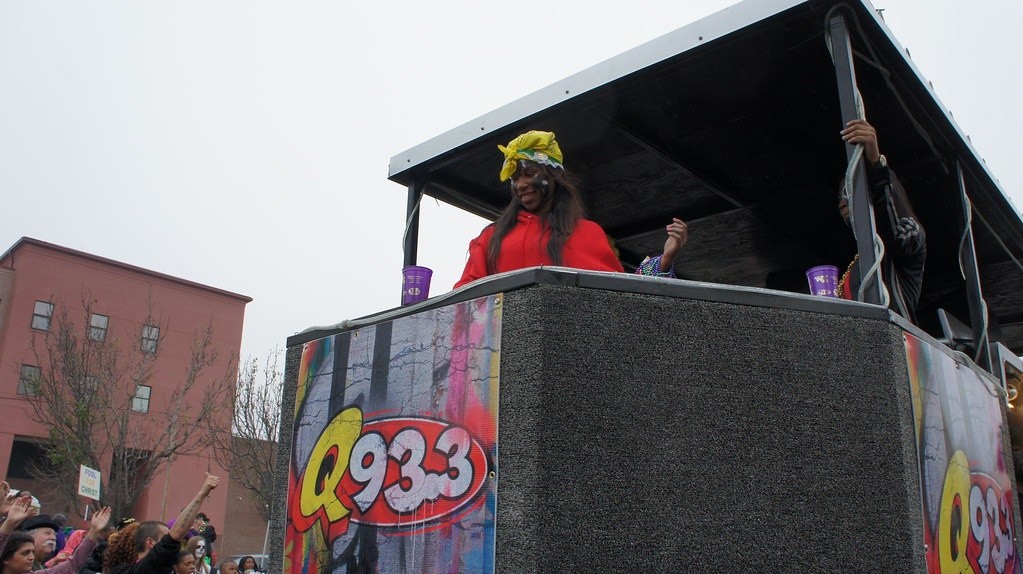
30 496 41 508
196 512 209 521
20 514 60 532
115 517 136 532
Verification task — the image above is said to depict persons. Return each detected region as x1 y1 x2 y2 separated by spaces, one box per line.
839 119 927 322
0 472 258 574
452 131 687 289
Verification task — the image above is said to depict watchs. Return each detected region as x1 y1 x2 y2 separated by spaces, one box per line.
869 154 887 168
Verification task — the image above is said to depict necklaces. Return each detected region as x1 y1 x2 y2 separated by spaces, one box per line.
636 255 676 278
837 254 859 299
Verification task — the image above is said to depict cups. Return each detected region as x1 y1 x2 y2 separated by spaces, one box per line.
400 266 434 305
805 265 840 300
